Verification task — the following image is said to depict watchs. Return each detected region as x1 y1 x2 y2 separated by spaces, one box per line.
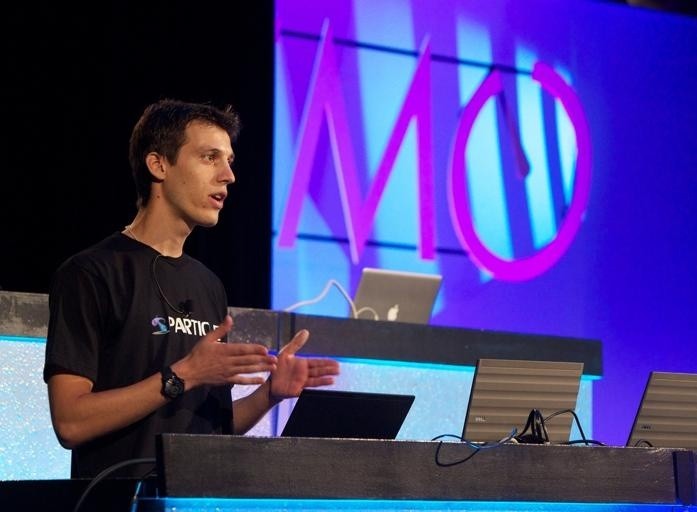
157 363 185 402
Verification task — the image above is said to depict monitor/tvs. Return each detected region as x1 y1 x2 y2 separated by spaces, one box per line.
463 359 585 445
281 387 415 439
351 268 442 323
625 372 697 448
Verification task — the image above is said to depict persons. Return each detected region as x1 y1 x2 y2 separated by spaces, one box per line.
42 98 340 478
